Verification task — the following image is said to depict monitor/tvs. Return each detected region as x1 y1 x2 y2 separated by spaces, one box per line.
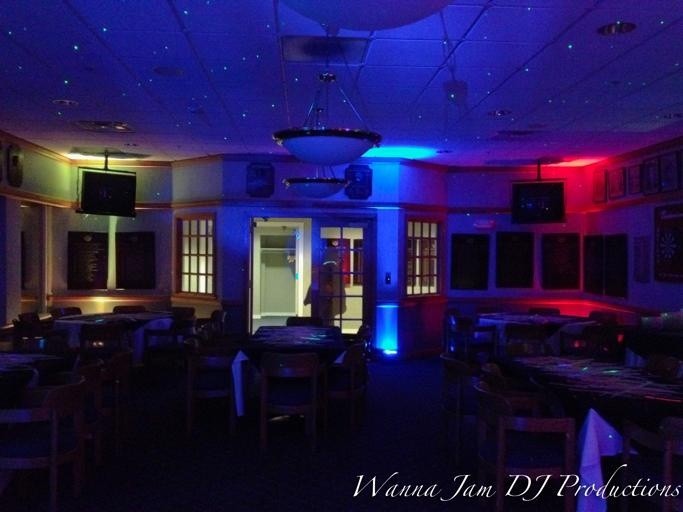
81 171 137 218
512 183 564 224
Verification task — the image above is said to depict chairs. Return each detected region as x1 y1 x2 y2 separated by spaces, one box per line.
1 300 683 511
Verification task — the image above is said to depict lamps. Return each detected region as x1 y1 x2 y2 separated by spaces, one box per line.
270 17 383 167
281 162 351 201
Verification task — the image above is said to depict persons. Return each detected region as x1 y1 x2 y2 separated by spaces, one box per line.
300 260 347 327
